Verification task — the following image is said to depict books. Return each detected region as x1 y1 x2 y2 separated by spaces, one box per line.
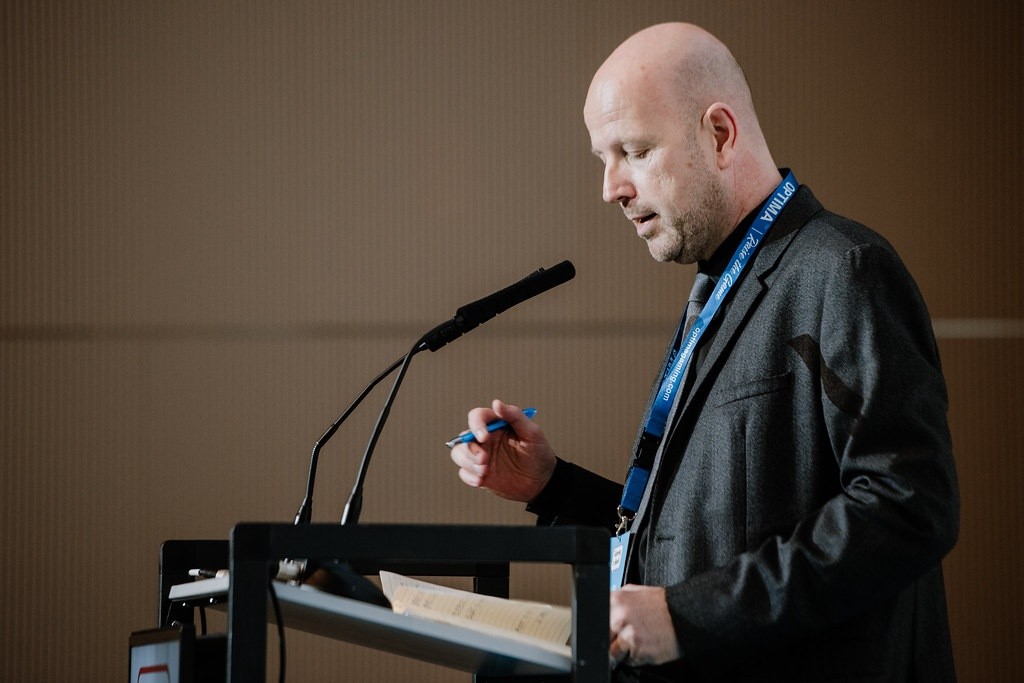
379 570 617 667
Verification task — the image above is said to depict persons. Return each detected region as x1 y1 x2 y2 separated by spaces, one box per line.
450 23 960 683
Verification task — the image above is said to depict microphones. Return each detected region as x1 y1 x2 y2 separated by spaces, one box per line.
289 260 577 609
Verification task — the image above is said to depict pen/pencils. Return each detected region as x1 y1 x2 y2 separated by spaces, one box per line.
443 405 539 455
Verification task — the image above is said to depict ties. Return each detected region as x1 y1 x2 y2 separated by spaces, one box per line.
627 273 713 585
